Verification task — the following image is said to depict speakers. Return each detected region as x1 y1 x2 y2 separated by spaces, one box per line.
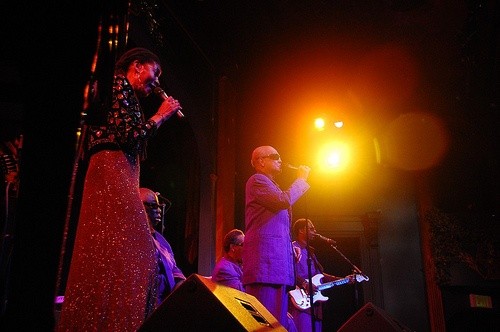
136 273 289 332
334 301 416 332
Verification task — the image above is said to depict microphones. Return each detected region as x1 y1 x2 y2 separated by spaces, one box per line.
314 233 336 244
285 162 302 169
153 87 184 121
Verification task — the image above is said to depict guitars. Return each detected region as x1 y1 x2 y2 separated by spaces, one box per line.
288 273 370 312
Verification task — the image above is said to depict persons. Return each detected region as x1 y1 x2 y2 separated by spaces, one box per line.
0 128 24 316
56 48 183 332
210 229 299 332
241 145 310 332
140 188 186 319
292 219 355 332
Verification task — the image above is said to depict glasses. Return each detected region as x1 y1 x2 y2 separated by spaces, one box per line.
142 202 164 210
256 154 279 160
304 227 314 231
